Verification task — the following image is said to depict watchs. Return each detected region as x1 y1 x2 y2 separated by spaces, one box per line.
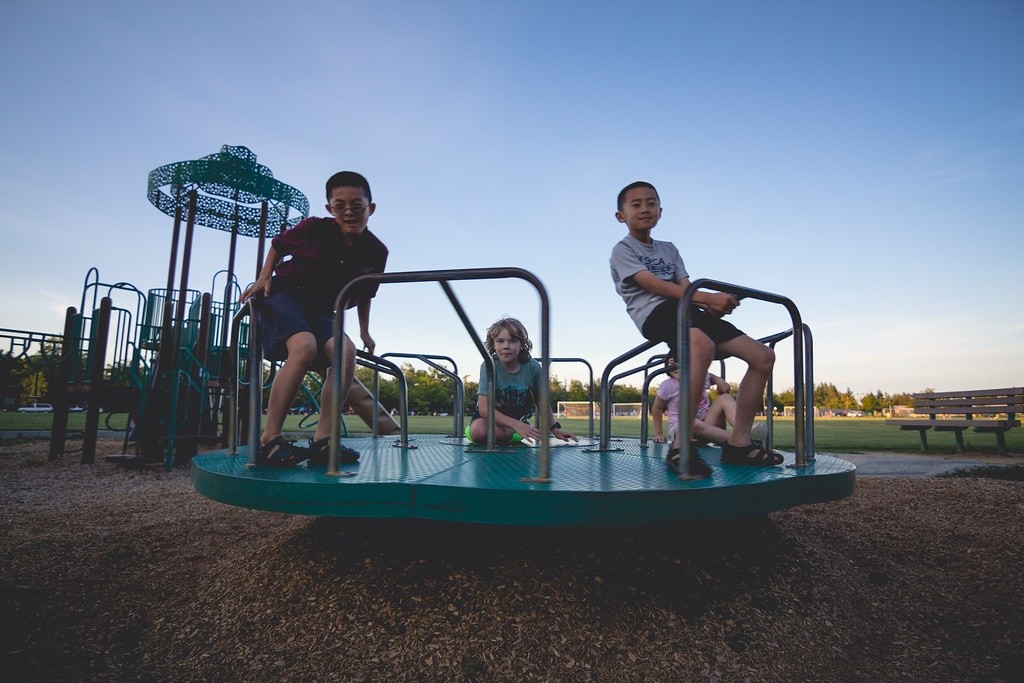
550 421 562 431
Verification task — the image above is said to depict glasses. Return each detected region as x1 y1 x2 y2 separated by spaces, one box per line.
331 202 371 215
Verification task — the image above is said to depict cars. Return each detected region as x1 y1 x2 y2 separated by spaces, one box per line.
846 411 865 417
552 411 564 417
18 403 54 414
71 405 84 413
438 410 449 416
464 410 474 416
831 412 846 417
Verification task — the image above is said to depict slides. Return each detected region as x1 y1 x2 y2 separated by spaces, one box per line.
346 371 402 436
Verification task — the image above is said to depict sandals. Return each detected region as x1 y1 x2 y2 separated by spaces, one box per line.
721 440 784 465
309 438 360 462
261 436 314 464
667 449 713 480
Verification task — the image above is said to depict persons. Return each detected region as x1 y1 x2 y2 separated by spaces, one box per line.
463 317 580 447
609 180 785 481
239 171 389 469
651 351 767 447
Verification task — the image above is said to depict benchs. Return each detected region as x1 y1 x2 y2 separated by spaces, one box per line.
884 386 1023 453
